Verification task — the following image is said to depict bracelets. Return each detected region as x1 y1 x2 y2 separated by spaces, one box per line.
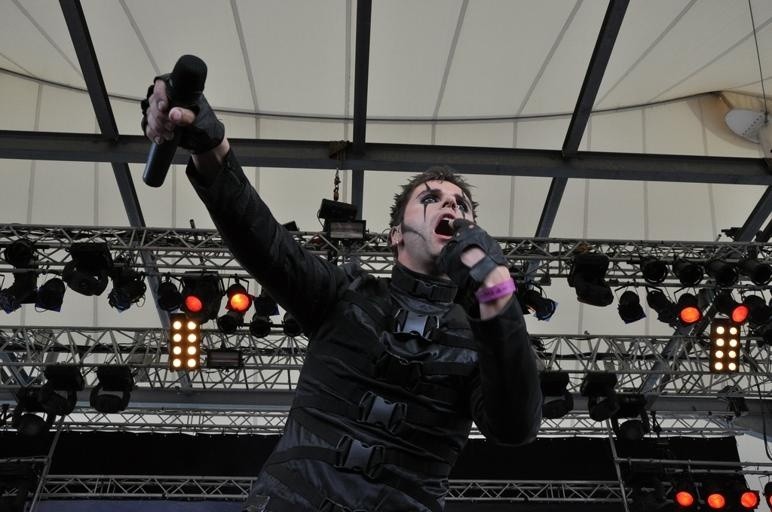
471 274 517 306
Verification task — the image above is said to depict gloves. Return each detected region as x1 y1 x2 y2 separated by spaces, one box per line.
140 73 225 156
433 217 508 297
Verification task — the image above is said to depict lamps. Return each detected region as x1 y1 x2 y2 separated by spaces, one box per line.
624 466 772 512
11 364 134 452
709 318 740 373
206 348 244 369
170 319 199 371
515 244 771 348
0 198 367 338
538 370 646 445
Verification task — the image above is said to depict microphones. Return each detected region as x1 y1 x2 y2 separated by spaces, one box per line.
142 55 208 188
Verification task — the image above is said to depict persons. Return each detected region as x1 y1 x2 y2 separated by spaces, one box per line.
139 67 544 512
